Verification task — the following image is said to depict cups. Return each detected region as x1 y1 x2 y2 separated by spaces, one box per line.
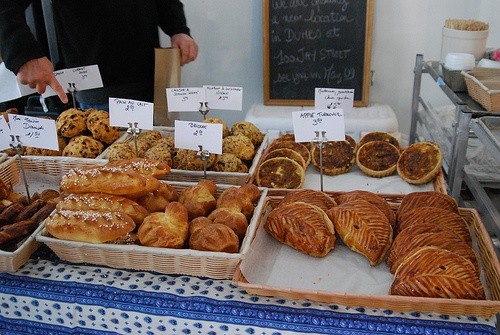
439 26 489 72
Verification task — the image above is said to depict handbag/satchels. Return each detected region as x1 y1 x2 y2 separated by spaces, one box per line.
25 92 74 121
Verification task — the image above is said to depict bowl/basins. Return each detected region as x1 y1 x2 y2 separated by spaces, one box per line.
477 58 500 69
444 53 475 70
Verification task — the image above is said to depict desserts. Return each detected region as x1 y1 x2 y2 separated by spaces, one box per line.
255 132 443 190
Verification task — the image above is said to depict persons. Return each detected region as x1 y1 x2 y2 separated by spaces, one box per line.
0 0 198 112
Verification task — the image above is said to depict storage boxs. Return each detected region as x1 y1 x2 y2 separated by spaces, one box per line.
245 103 401 139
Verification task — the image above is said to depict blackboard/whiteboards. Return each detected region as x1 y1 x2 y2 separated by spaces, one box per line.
263 0 374 106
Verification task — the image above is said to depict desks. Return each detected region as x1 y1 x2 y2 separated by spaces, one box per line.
0 246 500 335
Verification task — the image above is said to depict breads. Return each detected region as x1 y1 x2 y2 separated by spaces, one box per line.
0 107 122 160
0 179 62 251
44 159 261 255
266 187 487 302
105 117 264 172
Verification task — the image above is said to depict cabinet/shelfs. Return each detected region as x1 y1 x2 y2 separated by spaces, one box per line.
409 53 500 258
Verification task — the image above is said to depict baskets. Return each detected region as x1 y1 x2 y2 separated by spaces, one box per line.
36 180 268 279
0 155 108 273
461 71 500 115
255 138 447 203
231 195 500 318
97 130 269 186
